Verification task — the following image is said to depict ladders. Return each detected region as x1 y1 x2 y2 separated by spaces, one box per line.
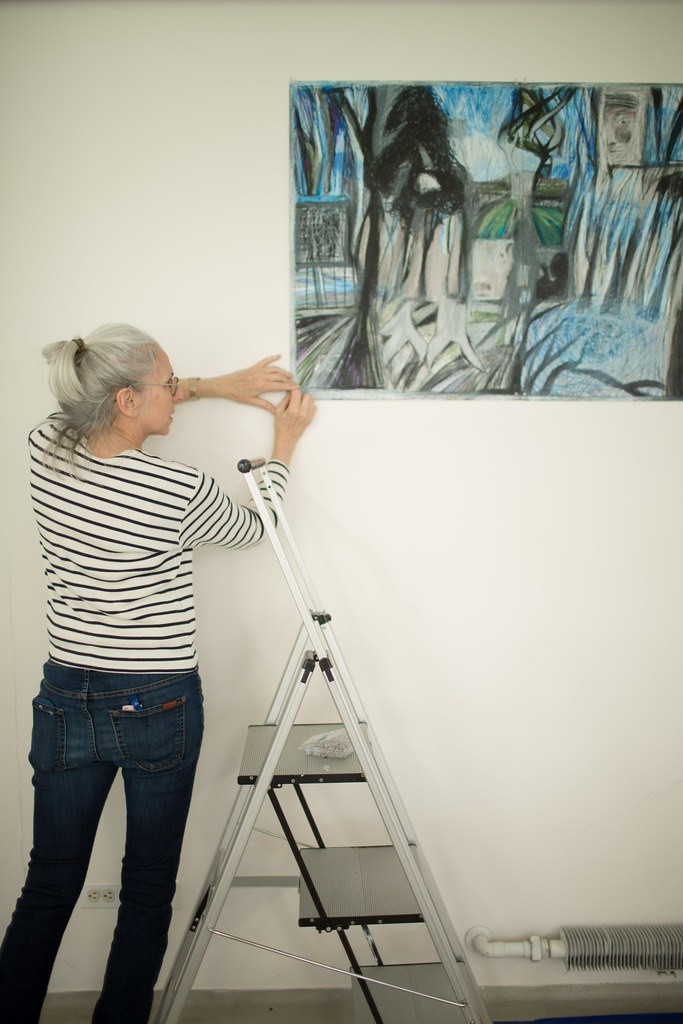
154 457 496 1023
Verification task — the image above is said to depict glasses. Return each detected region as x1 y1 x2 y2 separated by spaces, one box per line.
114 377 179 402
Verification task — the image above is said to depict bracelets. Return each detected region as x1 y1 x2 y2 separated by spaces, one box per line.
189 377 200 400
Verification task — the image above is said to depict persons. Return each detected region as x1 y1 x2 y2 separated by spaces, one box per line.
0 323 317 1024
